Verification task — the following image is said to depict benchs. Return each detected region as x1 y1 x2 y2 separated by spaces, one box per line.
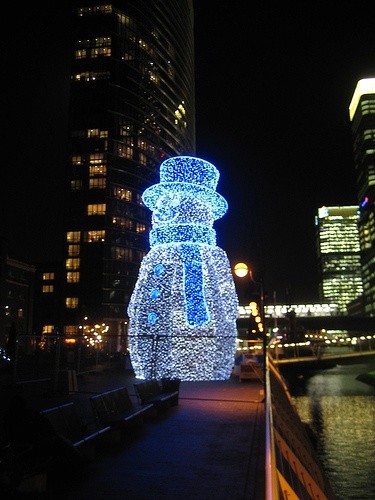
134 379 178 410
40 399 113 452
89 387 153 434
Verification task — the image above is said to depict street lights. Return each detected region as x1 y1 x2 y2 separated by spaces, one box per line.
232 262 269 405
